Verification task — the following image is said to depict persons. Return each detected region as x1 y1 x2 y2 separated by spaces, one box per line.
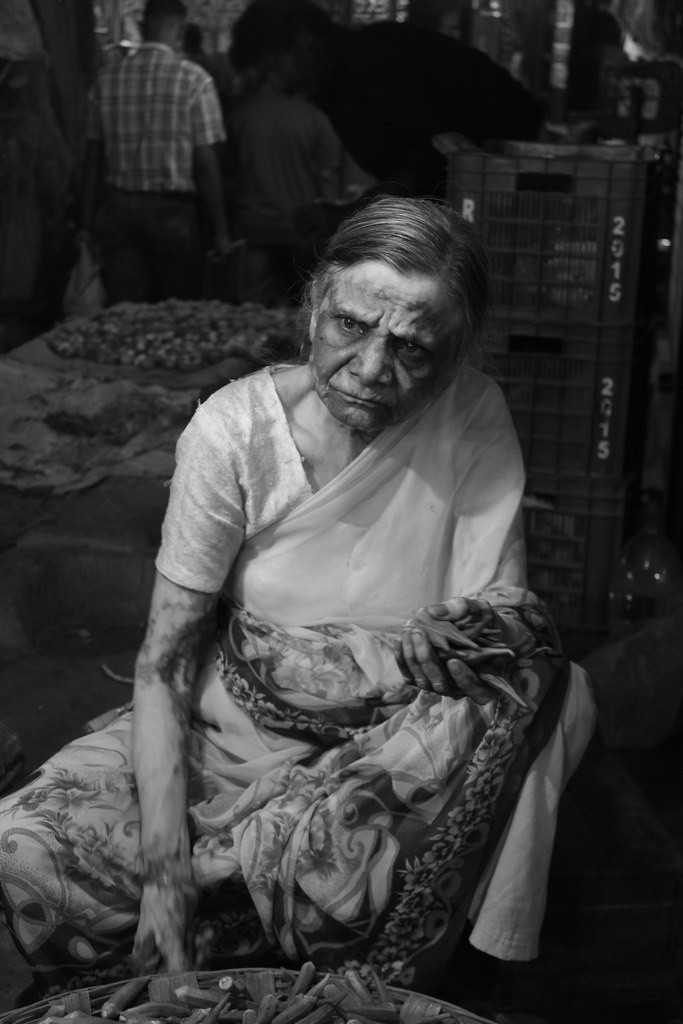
0 197 598 1005
78 0 547 309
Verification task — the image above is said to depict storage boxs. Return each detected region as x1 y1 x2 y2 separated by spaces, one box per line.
442 145 683 636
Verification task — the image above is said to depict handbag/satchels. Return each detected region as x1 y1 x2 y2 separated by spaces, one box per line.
61 241 107 316
